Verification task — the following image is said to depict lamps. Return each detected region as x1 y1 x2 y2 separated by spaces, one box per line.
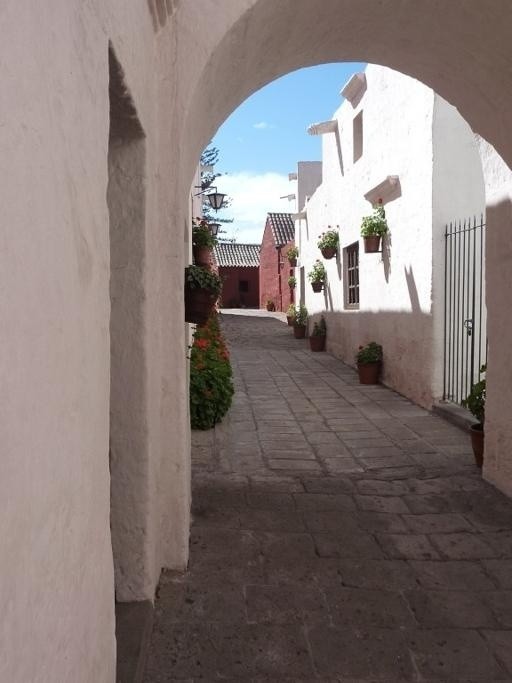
198 220 222 235
194 185 228 214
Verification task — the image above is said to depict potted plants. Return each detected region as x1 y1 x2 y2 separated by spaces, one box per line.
287 304 328 351
266 301 274 311
463 363 485 468
308 259 327 293
184 266 222 326
317 230 339 259
360 207 387 253
193 227 217 266
285 247 298 293
355 342 383 385
